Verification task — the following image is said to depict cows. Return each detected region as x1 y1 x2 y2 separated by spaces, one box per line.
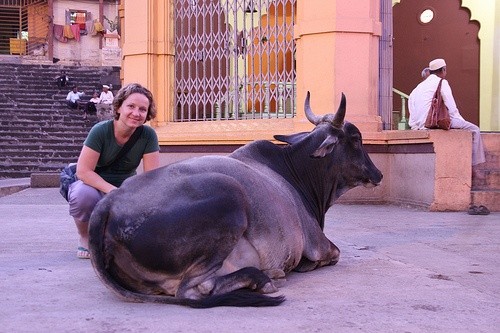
88 90 384 308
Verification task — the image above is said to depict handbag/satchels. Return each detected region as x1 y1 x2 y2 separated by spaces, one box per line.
59 162 83 202
424 78 452 130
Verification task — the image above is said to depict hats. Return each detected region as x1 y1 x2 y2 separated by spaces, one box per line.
429 58 447 71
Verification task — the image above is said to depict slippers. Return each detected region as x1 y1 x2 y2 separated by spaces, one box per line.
467 205 481 215
77 246 92 259
479 204 491 215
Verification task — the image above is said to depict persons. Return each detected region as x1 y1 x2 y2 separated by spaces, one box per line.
55 70 114 119
408 59 486 180
68 82 161 259
